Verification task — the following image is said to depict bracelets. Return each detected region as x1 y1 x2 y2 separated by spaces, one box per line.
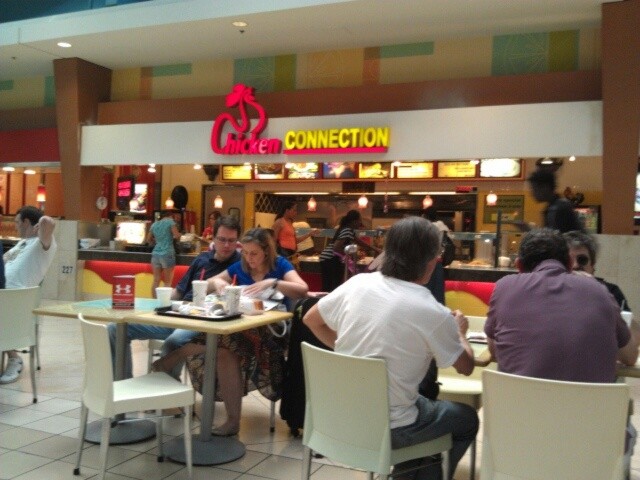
272 278 279 289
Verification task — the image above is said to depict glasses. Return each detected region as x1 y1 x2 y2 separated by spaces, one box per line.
215 237 238 245
571 255 593 266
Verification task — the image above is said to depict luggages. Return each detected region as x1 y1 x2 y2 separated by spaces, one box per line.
278 245 358 438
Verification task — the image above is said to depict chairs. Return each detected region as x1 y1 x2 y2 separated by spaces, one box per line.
73 311 196 478
0 286 41 405
300 339 453 479
475 369 630 480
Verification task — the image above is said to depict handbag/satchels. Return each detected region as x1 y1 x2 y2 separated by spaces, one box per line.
440 236 456 267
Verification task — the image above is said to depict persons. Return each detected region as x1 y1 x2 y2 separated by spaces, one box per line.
273 201 322 275
318 209 383 292
151 227 310 436
107 214 243 422
563 230 632 312
201 210 221 241
483 227 639 478
0 205 56 385
145 210 181 299
421 205 462 307
301 215 480 480
526 170 589 231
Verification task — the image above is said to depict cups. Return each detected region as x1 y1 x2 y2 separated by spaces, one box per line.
192 280 207 307
155 287 172 307
621 310 633 328
225 285 241 315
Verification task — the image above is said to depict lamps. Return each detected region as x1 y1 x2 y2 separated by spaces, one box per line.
358 196 368 209
35 185 47 202
307 197 316 212
422 196 433 210
214 195 223 208
164 197 176 209
485 192 498 206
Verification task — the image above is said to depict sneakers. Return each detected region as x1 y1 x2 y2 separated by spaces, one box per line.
1 358 23 383
